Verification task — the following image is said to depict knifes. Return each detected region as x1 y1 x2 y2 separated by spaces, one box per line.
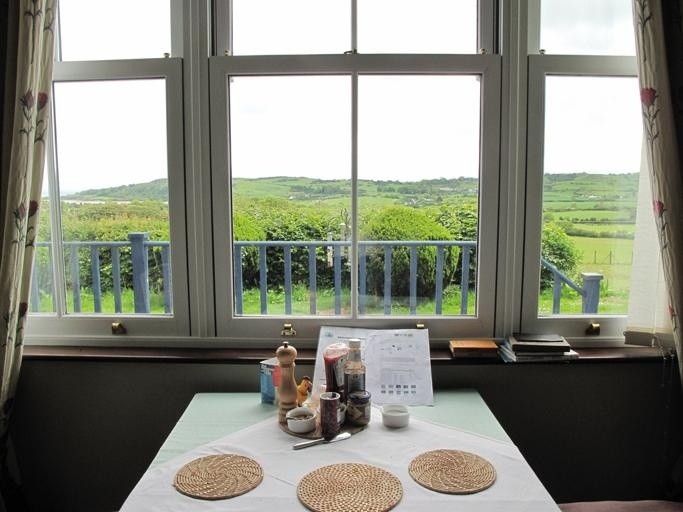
293 432 351 450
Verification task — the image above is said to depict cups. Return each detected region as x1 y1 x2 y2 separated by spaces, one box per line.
382 404 409 427
317 403 346 425
286 407 316 433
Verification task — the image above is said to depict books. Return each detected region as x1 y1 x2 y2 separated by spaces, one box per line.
498 334 579 364
449 340 498 359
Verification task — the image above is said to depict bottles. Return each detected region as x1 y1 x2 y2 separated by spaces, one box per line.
320 392 341 434
323 338 366 393
